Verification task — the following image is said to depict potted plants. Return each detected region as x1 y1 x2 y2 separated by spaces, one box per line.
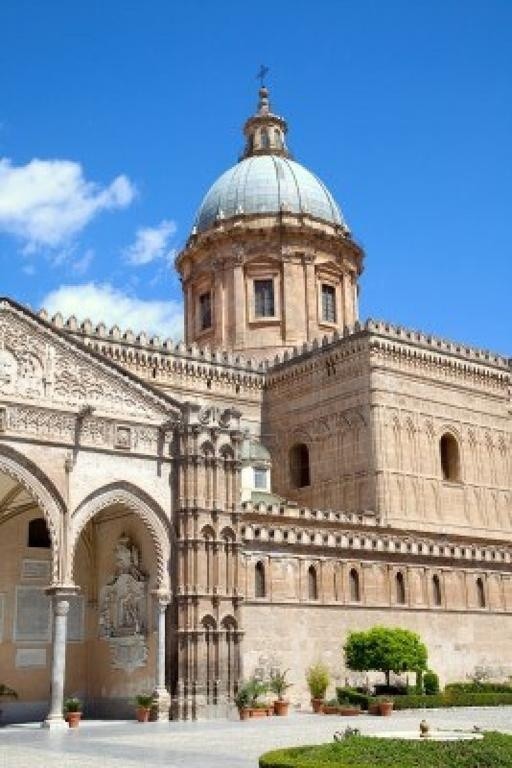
233 662 394 721
64 696 85 727
135 694 156 722
